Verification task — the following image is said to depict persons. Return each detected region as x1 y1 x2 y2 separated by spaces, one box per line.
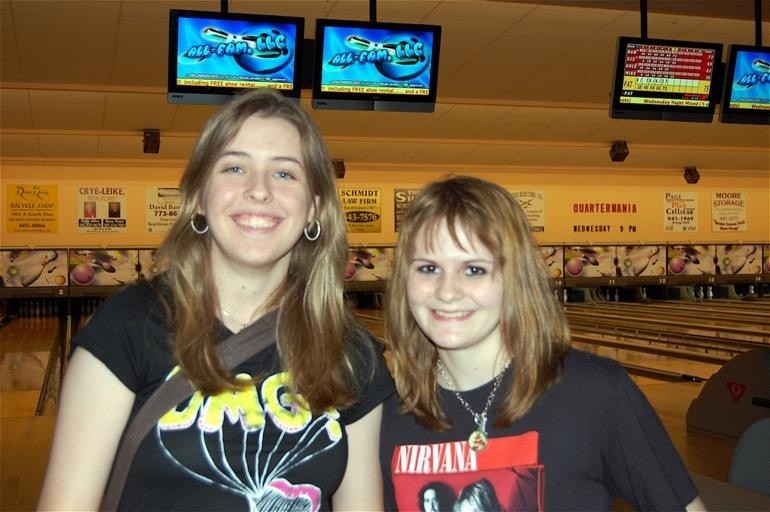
416 481 455 512
453 478 503 511
34 86 398 511
379 173 708 512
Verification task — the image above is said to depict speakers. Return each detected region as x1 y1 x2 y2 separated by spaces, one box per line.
331 160 345 178
684 168 700 183
144 129 160 153
610 143 630 162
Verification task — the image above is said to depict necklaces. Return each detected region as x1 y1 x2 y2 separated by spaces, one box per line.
218 306 252 331
432 350 519 451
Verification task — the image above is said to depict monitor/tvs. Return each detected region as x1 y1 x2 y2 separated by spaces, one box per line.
313 18 441 103
720 44 770 115
168 9 304 98
609 36 723 114
